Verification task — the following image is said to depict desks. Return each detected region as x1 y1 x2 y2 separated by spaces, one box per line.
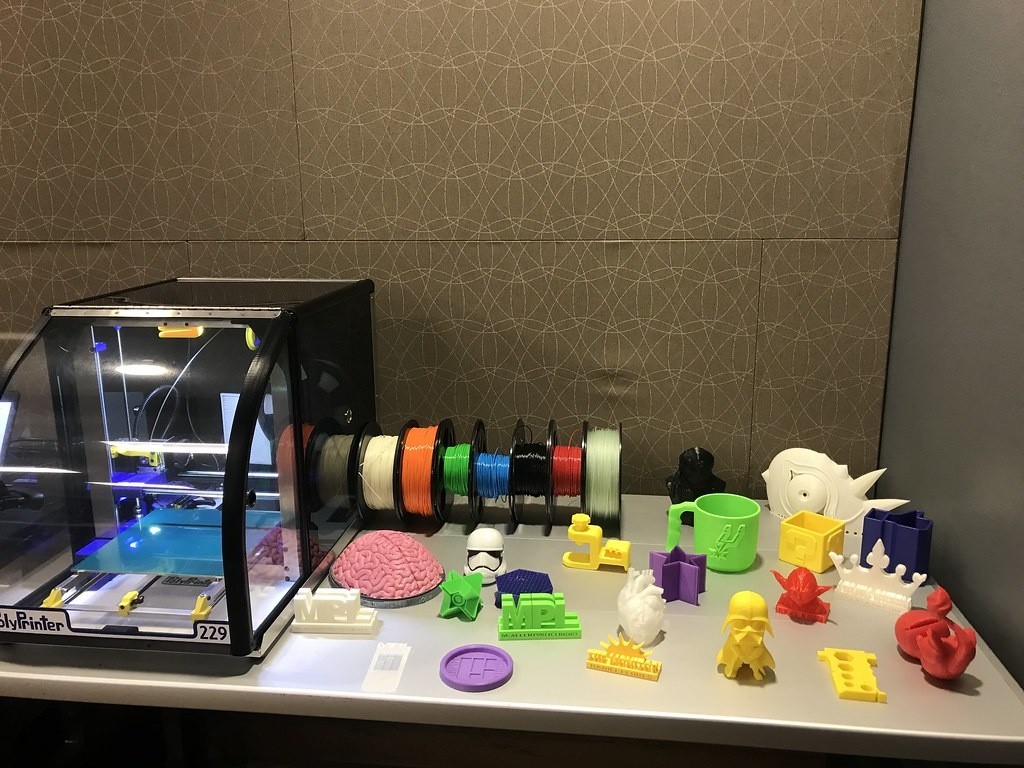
0 481 1024 763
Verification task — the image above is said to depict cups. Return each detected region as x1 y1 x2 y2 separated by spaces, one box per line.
667 493 760 573
